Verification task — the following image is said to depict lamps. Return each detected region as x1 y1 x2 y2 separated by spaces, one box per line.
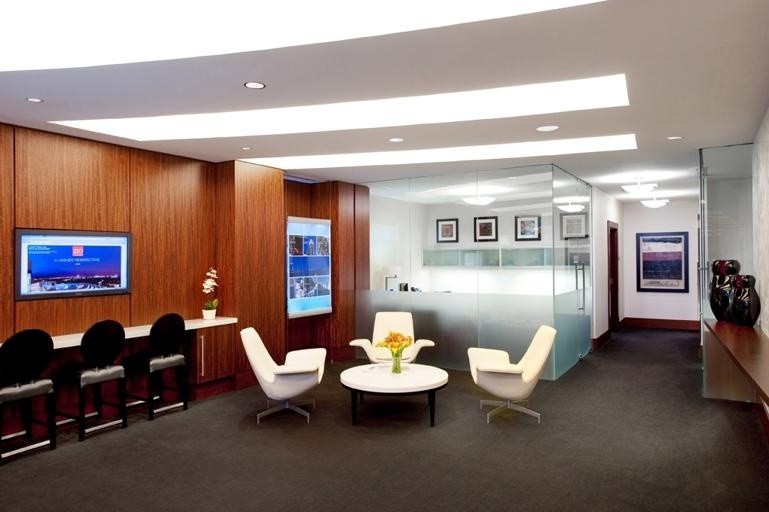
621 175 659 196
642 195 670 209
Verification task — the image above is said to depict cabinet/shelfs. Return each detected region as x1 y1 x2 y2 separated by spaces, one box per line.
195 328 241 397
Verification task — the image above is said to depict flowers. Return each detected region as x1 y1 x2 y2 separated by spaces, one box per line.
200 267 221 303
376 331 414 353
200 308 222 322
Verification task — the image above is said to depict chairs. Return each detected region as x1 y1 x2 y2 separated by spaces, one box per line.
347 307 435 364
76 319 130 442
0 329 66 469
239 324 325 424
467 326 562 425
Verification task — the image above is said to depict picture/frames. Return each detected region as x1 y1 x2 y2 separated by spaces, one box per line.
435 218 460 243
474 216 499 243
559 212 590 240
515 215 541 242
635 232 689 293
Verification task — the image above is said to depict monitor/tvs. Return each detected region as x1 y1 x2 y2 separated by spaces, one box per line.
12 226 133 302
400 282 408 291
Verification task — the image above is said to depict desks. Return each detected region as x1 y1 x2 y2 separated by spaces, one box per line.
0 314 251 402
702 318 769 430
125 313 246 402
339 366 448 426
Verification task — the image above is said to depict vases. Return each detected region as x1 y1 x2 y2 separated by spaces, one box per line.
393 350 402 373
